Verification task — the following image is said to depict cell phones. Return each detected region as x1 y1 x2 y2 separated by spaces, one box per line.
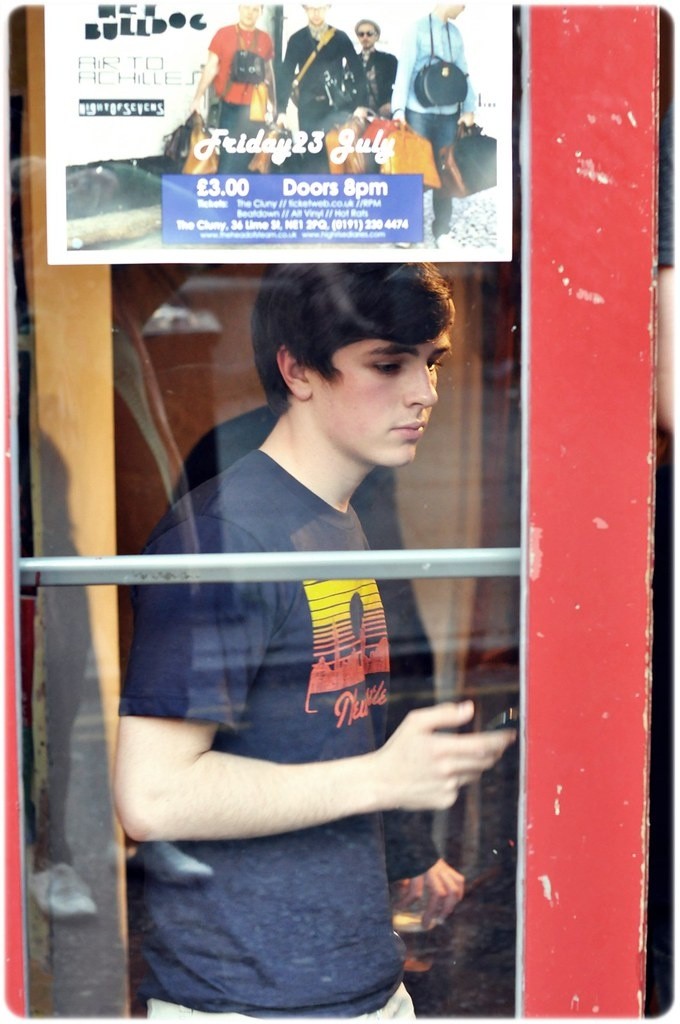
484 709 520 731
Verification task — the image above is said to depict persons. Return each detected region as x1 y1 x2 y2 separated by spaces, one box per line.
114 263 516 1021
392 4 476 247
20 146 214 919
275 5 368 175
354 19 397 114
189 5 277 175
